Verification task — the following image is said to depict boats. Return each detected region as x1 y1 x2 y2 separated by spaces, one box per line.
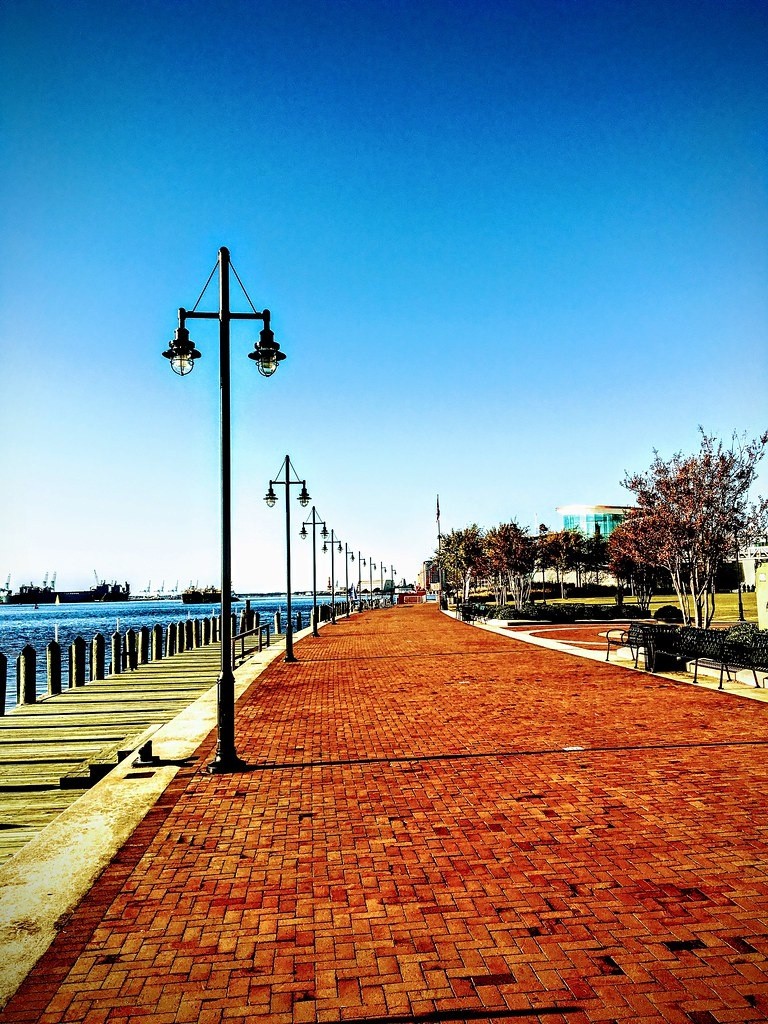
128 580 181 601
0 570 131 604
182 578 240 604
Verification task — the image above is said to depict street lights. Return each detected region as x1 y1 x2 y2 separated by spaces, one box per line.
381 562 387 598
161 247 287 774
263 455 312 661
299 506 329 637
358 551 366 613
321 529 343 625
369 557 376 609
345 543 355 618
391 565 396 607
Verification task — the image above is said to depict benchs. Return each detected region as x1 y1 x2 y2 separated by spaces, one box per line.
651 627 733 683
718 634 768 690
605 622 679 669
